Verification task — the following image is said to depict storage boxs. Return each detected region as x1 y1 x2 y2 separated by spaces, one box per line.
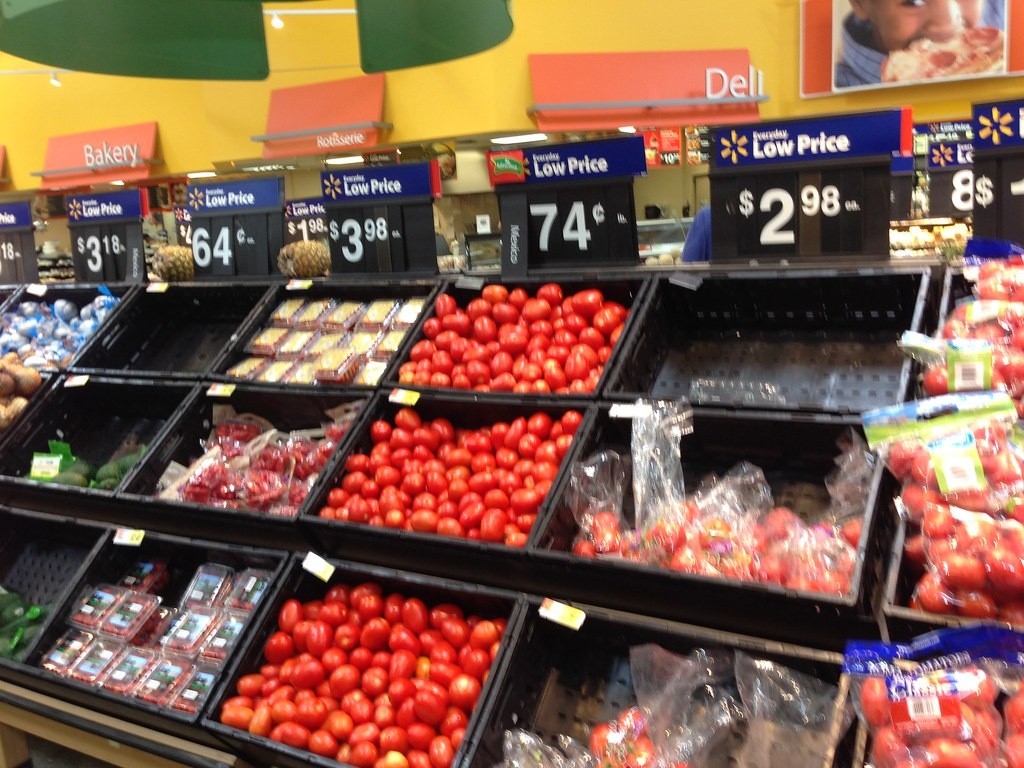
380 273 653 400
65 281 283 381
881 513 977 644
0 505 113 663
21 524 290 723
206 274 444 391
0 280 137 374
114 380 374 552
0 284 22 312
0 372 198 519
601 265 940 415
937 263 979 331
0 373 53 447
527 399 903 625
200 553 526 767
297 387 596 595
458 593 858 768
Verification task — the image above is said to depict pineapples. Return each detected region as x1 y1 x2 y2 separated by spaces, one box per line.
277 241 330 278
150 245 196 282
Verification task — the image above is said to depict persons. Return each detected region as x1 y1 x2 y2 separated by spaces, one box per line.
832 0 1005 88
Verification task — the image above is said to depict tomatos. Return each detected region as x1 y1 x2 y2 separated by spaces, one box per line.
921 255 1024 423
399 283 632 394
320 408 583 546
571 500 860 597
591 707 693 768
42 561 272 715
219 582 508 768
858 664 1024 768
888 421 1024 630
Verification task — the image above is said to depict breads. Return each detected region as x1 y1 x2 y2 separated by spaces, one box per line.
880 26 1005 85
